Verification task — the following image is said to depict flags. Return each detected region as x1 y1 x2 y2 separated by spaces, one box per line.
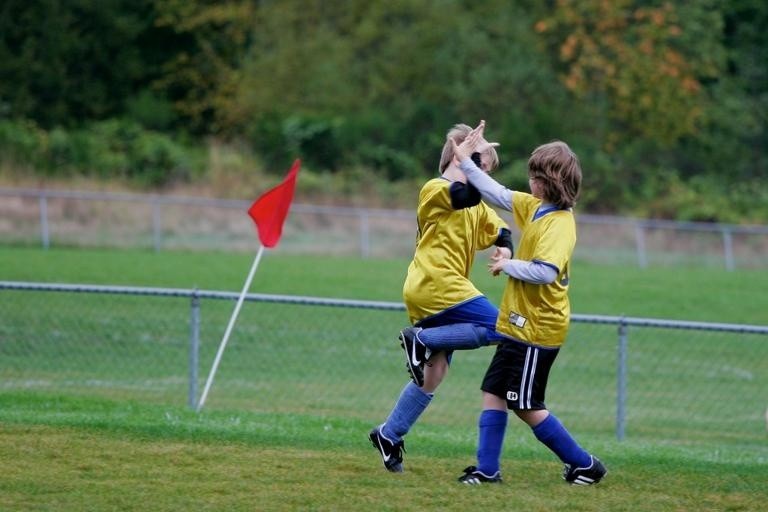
247 159 297 250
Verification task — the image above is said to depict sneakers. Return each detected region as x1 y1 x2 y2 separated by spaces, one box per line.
455 464 503 487
368 421 406 474
558 453 609 488
400 325 430 388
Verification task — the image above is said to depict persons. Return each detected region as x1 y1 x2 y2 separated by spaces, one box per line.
449 135 609 488
366 118 516 473
